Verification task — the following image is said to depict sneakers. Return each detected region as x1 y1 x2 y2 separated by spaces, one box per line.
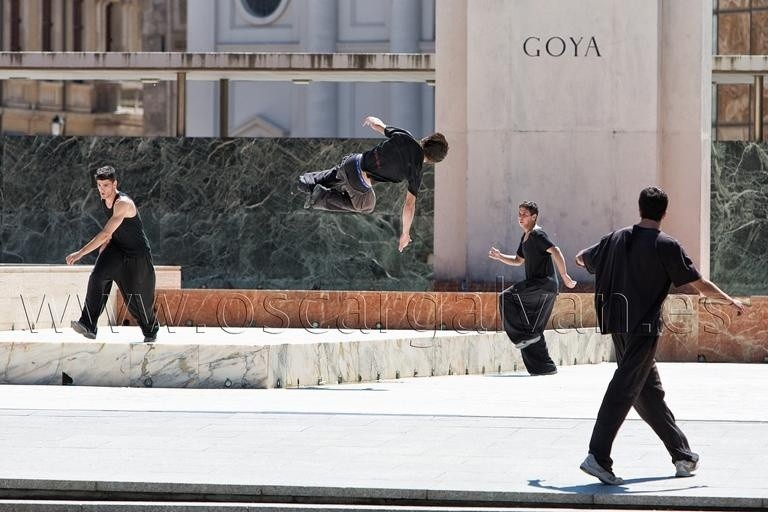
296 175 328 210
514 334 541 349
579 452 624 485
142 332 158 343
530 368 558 376
673 458 702 478
71 319 98 340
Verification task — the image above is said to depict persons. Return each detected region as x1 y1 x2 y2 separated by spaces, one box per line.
297 116 449 253
66 165 160 342
488 200 578 376
574 186 745 486
